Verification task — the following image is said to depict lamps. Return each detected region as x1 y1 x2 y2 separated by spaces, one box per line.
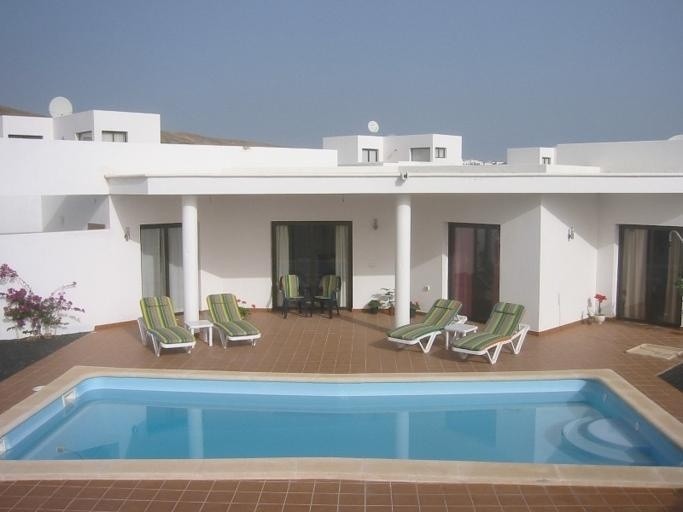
568 226 575 238
121 227 133 241
371 218 379 230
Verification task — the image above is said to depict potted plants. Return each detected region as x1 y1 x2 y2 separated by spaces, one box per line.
380 287 395 316
366 298 380 315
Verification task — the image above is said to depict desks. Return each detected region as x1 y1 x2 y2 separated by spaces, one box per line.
186 317 216 350
444 322 480 352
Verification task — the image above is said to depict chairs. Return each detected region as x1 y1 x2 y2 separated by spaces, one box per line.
135 293 198 358
452 300 531 366
310 273 344 320
277 271 314 321
206 290 263 350
385 294 468 355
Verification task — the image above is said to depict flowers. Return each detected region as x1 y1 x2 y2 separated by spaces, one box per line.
593 294 610 314
410 300 421 310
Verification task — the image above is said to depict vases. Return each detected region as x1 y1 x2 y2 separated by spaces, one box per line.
410 308 416 318
594 315 607 326
